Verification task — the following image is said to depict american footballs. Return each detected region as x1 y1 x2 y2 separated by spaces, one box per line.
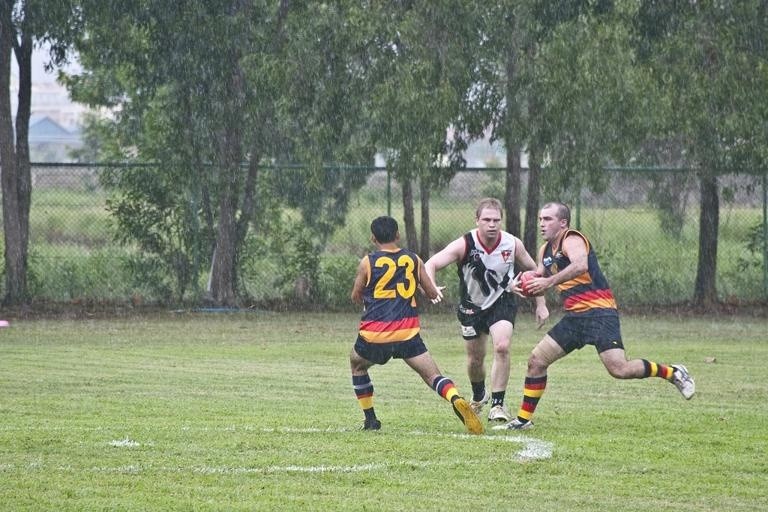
519 271 543 296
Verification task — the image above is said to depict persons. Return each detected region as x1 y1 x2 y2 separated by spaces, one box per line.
424 196 550 422
350 216 484 435
490 200 696 431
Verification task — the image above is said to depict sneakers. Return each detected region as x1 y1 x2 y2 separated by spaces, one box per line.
453 392 532 433
362 420 381 429
670 365 695 400
